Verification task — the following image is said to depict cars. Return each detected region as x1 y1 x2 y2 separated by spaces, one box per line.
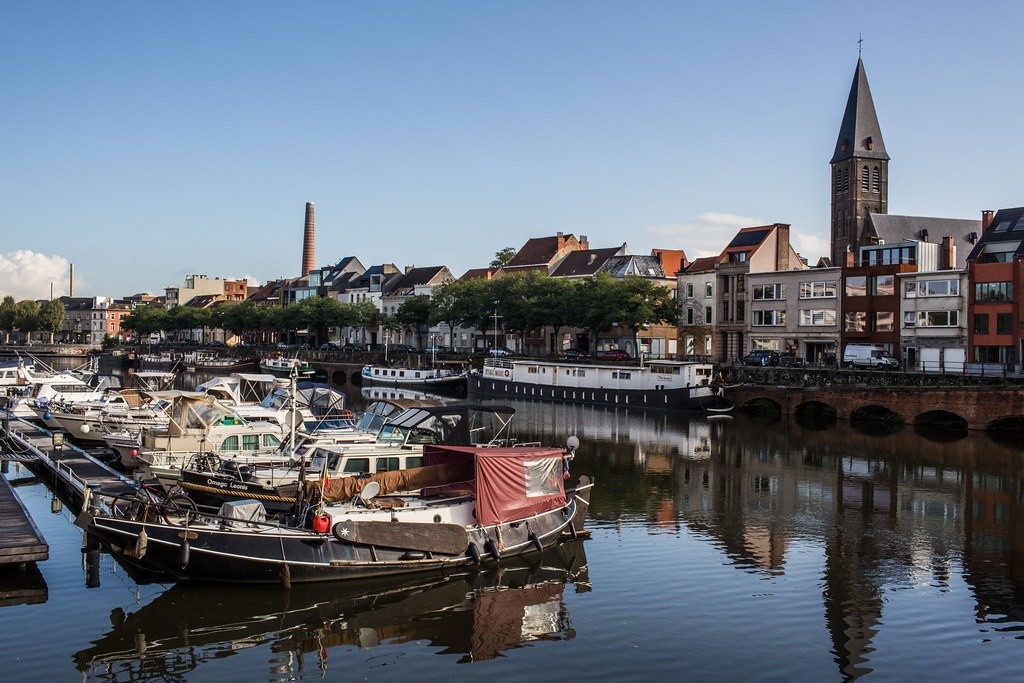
425 345 446 354
489 347 514 358
564 348 591 360
211 341 224 347
277 342 294 350
603 350 629 361
321 343 338 350
741 349 779 365
298 342 315 350
396 344 418 353
342 344 362 352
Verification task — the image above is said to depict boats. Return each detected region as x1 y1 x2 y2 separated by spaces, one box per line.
72 530 593 683
361 332 470 384
182 349 254 370
142 351 183 366
259 356 316 371
74 403 595 585
361 379 464 403
0 365 579 521
467 358 735 412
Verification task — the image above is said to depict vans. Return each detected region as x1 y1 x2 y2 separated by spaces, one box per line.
844 345 898 370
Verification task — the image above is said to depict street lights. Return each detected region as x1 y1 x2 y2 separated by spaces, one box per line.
295 327 298 349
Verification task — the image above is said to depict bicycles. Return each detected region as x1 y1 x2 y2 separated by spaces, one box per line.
111 476 197 527
